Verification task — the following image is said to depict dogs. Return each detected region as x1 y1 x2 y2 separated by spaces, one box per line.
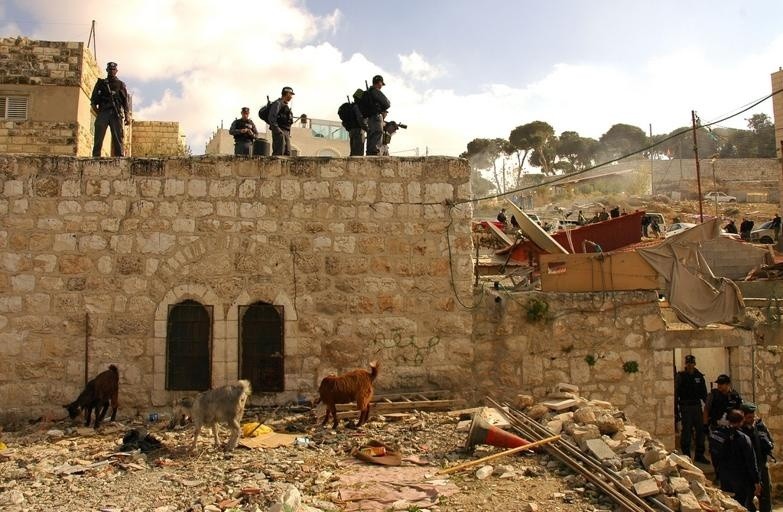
61 363 121 429
173 379 253 456
318 359 382 429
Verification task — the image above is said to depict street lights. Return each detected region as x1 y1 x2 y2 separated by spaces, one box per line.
709 151 721 219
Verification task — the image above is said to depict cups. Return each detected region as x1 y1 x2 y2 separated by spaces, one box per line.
295 438 309 444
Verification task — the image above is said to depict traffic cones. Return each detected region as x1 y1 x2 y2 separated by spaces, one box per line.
462 408 538 456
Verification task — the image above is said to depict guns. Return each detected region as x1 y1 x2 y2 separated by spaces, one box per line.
102 79 122 119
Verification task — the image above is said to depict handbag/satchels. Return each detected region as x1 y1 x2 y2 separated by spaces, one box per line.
338 103 358 130
354 88 380 118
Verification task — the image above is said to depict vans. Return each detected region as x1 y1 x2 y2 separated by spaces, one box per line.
640 212 667 237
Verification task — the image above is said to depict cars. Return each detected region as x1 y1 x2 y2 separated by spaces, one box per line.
555 218 579 231
750 221 783 244
704 191 738 203
665 221 697 238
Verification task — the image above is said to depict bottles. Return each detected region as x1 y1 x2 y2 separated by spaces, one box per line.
241 487 261 494
145 413 171 420
359 446 388 456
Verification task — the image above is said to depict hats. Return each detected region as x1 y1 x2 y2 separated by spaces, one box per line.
740 403 757 413
685 355 695 363
282 87 295 95
107 62 117 68
715 374 730 384
242 107 249 111
373 76 385 86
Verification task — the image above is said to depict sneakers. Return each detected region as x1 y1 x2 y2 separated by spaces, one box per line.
694 457 710 464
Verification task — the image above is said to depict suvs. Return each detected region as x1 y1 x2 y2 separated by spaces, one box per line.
525 213 541 229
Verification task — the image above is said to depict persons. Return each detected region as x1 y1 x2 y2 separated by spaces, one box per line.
673 354 776 511
770 214 781 240
267 87 295 156
90 62 131 157
740 218 750 242
721 220 737 233
511 207 523 229
497 208 508 228
577 206 661 239
229 107 258 155
341 76 397 157
513 191 533 210
